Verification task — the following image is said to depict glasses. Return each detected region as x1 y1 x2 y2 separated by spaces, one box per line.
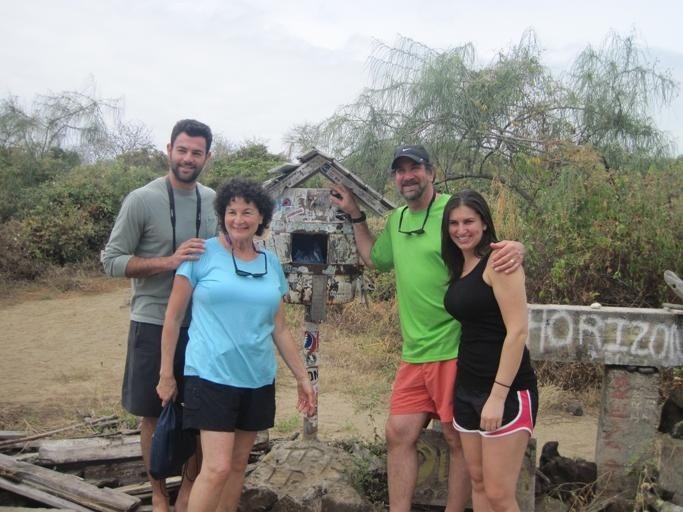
232 248 267 277
398 205 430 235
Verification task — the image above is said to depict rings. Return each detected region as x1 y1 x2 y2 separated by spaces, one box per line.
510 259 516 265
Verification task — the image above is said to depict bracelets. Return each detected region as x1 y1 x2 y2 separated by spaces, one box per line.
494 380 511 391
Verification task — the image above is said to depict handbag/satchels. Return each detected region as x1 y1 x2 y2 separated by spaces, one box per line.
149 399 196 479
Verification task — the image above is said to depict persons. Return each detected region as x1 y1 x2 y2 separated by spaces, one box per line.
441 189 538 512
155 179 317 512
100 120 221 511
330 146 526 512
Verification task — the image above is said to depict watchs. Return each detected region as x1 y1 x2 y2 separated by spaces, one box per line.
351 211 366 223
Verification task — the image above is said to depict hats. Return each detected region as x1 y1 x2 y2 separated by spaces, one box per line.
391 144 429 169
